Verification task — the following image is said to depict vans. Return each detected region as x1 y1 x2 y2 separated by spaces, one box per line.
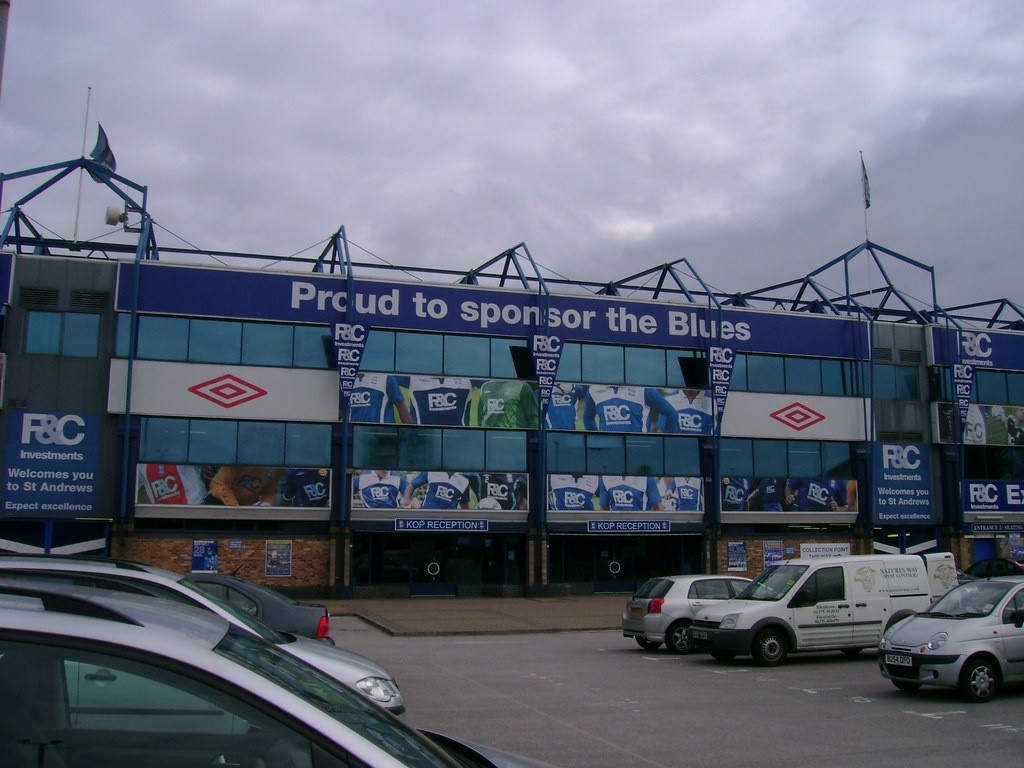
690 550 962 666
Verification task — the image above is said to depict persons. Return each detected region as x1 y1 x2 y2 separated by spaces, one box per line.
137 464 329 508
722 478 849 513
544 384 717 434
353 469 526 510
350 372 539 428
547 475 704 511
964 404 1024 446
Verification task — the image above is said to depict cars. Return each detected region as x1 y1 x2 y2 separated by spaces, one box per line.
962 556 1024 593
879 573 1023 701
183 575 335 644
1 580 565 768
0 552 406 735
955 566 991 601
622 572 781 653
